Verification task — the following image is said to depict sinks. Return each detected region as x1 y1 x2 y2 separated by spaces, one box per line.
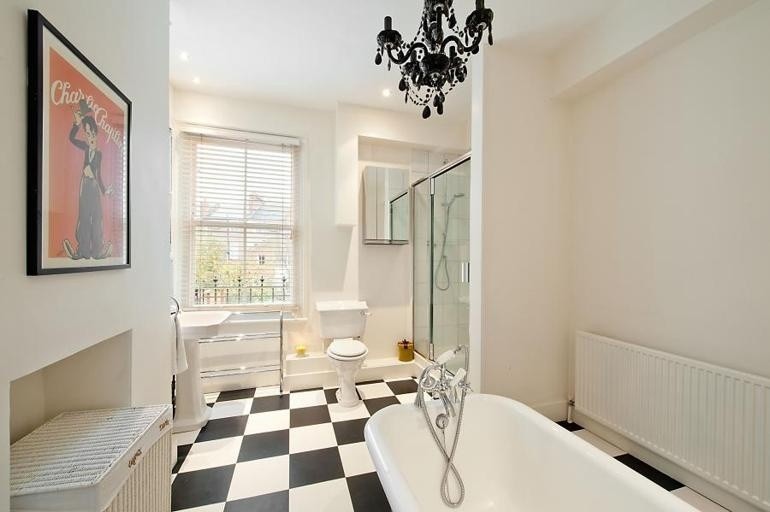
180 311 234 339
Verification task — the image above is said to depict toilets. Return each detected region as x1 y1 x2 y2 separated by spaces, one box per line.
316 299 371 406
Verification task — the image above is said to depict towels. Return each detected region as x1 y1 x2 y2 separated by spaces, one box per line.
170 313 188 374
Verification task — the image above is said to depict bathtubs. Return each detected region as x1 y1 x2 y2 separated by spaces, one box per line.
364 394 700 512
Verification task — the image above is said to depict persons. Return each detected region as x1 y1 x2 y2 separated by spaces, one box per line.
59 98 117 261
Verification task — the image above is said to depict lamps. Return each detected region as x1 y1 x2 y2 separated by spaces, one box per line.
374 0 493 119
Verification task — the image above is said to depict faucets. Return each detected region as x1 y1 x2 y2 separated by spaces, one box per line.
440 391 456 418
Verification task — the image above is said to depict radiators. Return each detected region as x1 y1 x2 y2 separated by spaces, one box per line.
568 329 770 512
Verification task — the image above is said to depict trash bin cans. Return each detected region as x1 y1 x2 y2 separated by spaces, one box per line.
399 339 414 361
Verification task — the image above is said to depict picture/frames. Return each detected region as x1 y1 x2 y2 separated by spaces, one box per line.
24 9 135 275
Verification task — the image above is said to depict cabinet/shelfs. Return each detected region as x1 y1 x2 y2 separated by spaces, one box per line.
9 405 173 512
